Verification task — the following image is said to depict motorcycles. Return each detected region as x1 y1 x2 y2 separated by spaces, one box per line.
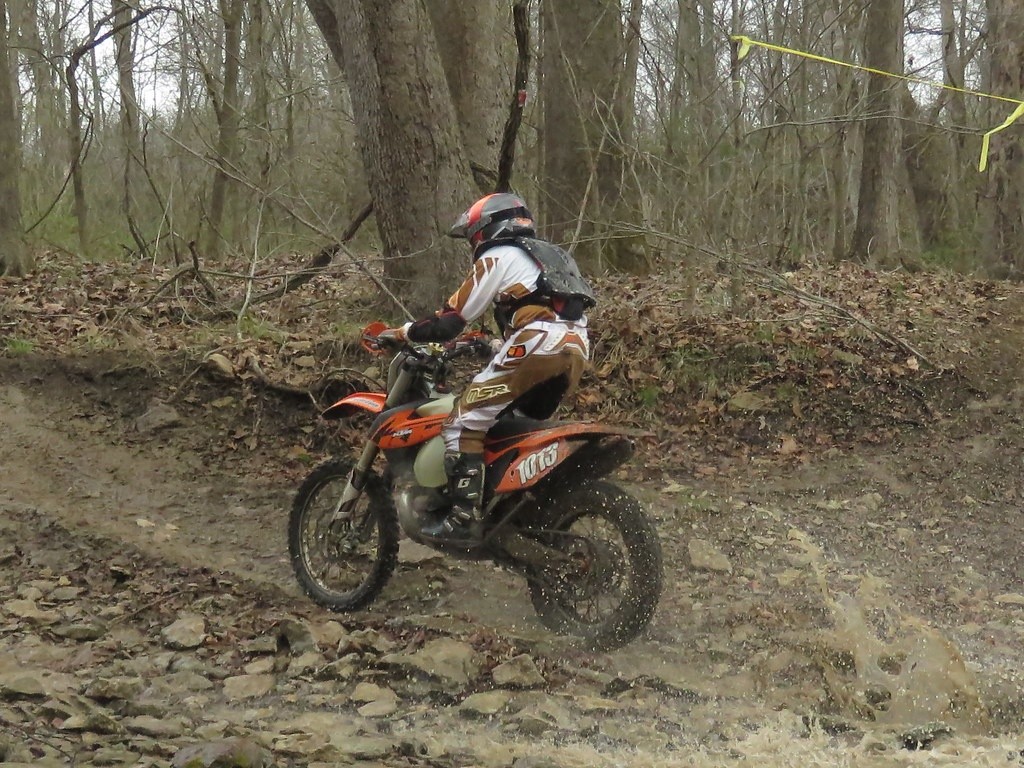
286 319 665 653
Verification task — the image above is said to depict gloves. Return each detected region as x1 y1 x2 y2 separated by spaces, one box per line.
489 334 507 354
377 322 413 350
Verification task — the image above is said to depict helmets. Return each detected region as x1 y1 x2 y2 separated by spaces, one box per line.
446 192 537 255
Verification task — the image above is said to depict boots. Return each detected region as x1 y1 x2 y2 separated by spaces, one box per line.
418 461 485 549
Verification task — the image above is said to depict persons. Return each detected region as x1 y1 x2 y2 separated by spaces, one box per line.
377 192 590 546
